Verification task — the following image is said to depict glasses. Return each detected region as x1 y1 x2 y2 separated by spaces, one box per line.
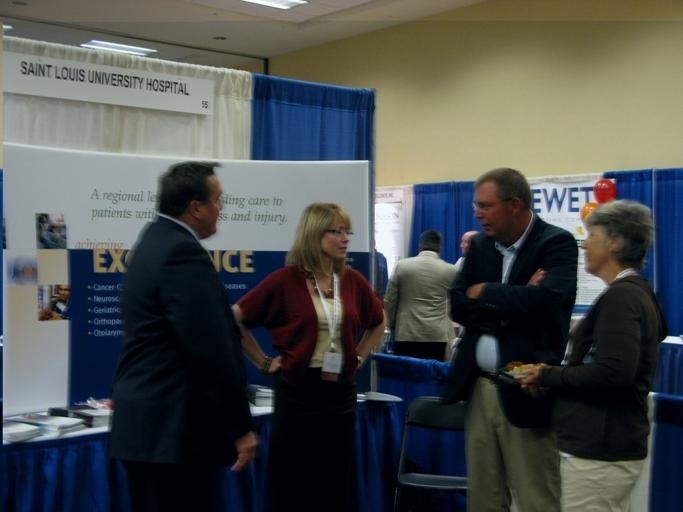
472 196 521 208
325 230 354 237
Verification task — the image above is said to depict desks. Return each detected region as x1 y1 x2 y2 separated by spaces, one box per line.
0 388 402 509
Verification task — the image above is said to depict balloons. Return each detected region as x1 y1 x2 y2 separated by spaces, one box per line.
594 180 617 203
580 202 598 221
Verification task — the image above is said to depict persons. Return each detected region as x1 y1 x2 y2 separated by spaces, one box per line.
383 229 459 362
438 167 578 512
109 160 260 512
40 285 70 320
375 240 388 299
455 230 480 272
520 199 669 512
231 203 386 512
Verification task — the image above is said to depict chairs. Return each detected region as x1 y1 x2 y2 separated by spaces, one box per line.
390 395 469 511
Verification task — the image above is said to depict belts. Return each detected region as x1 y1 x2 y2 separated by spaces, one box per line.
479 370 495 379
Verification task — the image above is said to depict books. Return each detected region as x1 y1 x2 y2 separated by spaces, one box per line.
2 405 112 444
248 383 274 417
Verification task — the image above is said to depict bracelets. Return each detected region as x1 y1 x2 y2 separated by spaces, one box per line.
260 356 272 373
356 354 367 366
